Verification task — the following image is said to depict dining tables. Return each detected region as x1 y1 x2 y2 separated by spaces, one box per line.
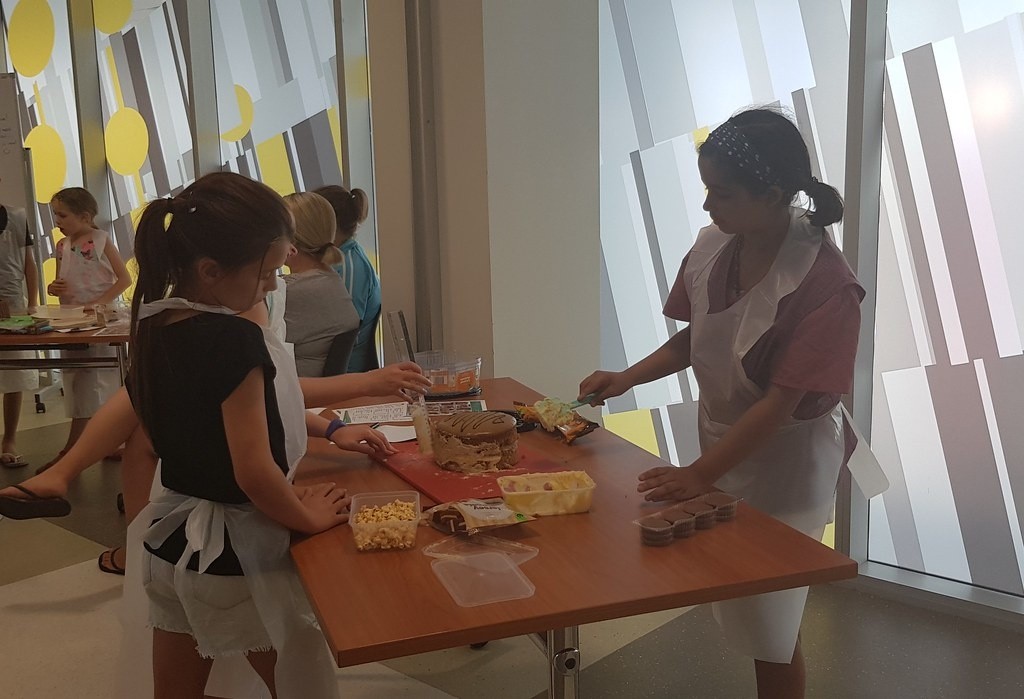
0 304 132 385
289 375 859 698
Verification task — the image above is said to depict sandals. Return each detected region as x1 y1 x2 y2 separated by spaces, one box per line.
36 450 67 474
105 447 125 462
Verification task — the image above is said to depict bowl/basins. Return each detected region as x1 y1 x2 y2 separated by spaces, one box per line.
496 470 597 515
46 303 85 321
348 490 420 551
402 352 482 399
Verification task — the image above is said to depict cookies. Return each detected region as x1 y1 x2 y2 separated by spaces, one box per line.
641 493 738 547
435 509 465 532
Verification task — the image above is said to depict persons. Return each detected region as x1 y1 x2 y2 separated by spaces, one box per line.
1 200 39 468
119 173 350 698
575 108 865 698
36 186 132 483
237 268 432 457
314 184 383 380
0 190 360 574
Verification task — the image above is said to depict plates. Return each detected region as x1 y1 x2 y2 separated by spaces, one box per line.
485 409 537 431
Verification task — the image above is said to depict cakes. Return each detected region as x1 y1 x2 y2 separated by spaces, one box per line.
431 409 520 470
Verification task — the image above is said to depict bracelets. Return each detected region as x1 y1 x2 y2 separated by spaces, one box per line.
47 284 54 296
323 418 346 442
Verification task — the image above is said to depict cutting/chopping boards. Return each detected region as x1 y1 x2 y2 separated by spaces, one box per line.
39 317 98 332
367 441 573 505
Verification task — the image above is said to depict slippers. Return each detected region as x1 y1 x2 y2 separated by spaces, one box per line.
1 484 73 521
1 453 29 467
99 546 126 576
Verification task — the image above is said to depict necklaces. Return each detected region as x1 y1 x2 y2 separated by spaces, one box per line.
729 232 747 300
